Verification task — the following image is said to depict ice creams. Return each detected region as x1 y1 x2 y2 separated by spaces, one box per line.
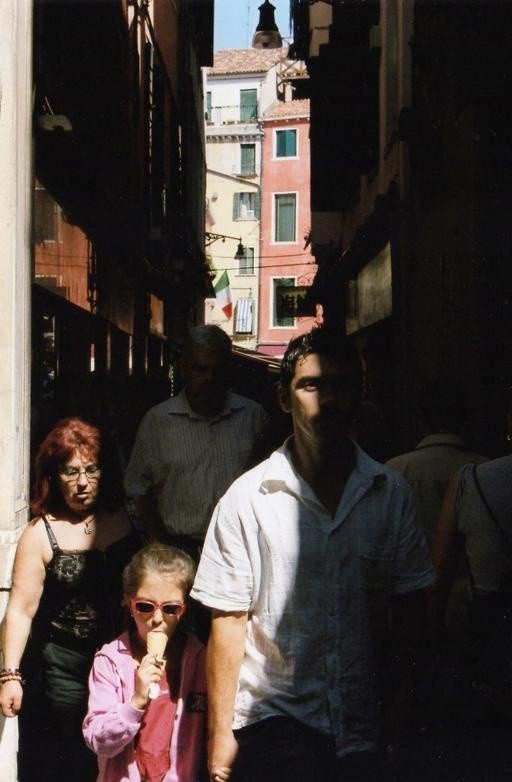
147 630 168 667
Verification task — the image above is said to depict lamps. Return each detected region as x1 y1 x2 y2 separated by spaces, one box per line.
252 1 282 49
203 232 246 260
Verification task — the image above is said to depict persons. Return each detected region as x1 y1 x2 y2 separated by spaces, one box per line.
188 324 441 782
0 414 141 781
425 384 512 782
32 358 402 514
81 543 206 782
382 390 488 736
120 324 274 782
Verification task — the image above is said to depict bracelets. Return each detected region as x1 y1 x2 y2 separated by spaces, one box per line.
0 668 23 683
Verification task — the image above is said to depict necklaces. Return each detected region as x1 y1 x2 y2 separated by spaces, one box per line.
67 505 99 535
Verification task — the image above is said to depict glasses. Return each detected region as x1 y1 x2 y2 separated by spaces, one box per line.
130 598 186 616
60 467 100 481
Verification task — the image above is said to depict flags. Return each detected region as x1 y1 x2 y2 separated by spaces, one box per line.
212 268 235 320
